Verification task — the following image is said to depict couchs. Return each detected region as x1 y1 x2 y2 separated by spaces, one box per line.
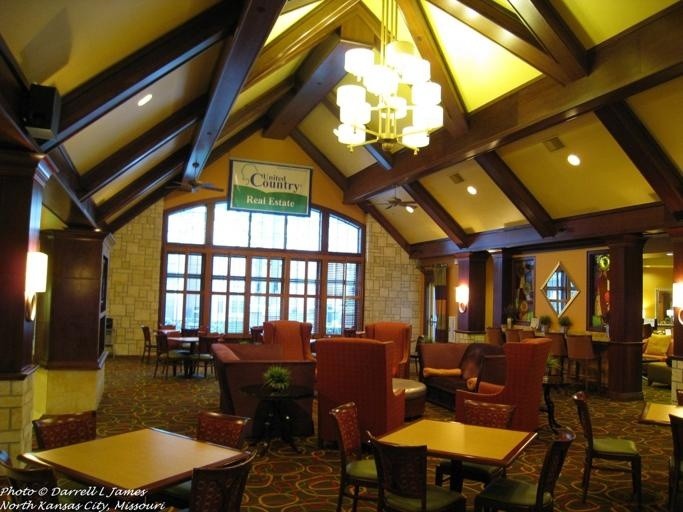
416 324 551 430
646 361 671 388
209 340 292 417
263 320 315 368
641 333 673 374
362 322 413 381
311 337 410 447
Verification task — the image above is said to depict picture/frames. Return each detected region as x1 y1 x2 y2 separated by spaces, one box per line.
509 256 537 328
586 250 610 335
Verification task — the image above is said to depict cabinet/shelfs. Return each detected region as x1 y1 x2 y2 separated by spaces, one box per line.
39 225 119 371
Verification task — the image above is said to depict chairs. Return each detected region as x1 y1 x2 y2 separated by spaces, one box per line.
327 400 379 512
564 332 603 392
364 428 466 511
189 333 217 379
570 390 642 505
667 413 683 511
1 460 60 512
518 330 534 344
484 327 502 345
433 399 517 493
541 332 565 388
31 408 100 449
154 449 258 512
157 324 183 375
250 323 263 339
152 331 186 381
474 426 576 511
193 409 253 450
504 328 522 343
141 326 157 365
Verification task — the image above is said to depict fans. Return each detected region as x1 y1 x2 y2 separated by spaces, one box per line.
163 162 224 195
366 185 418 217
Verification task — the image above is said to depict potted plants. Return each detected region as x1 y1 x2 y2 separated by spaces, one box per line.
539 314 551 333
557 314 572 338
262 362 294 387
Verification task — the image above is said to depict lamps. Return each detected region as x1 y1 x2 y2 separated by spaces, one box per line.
466 184 477 196
331 0 444 155
136 93 153 106
23 248 48 323
400 200 414 217
453 282 471 313
665 308 671 319
671 280 683 325
565 153 581 164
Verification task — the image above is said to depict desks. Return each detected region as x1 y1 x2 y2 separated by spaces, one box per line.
376 417 539 492
15 426 253 496
241 385 318 458
166 336 199 373
389 377 426 420
638 400 682 427
